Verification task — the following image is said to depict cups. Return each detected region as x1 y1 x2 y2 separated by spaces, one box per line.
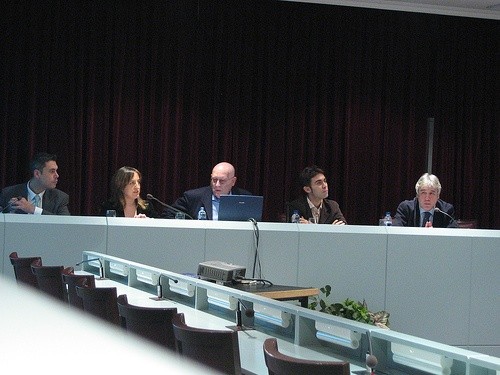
107 210 116 217
175 213 185 219
308 218 316 224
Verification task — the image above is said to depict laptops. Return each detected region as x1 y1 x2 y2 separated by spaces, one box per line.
218 196 263 222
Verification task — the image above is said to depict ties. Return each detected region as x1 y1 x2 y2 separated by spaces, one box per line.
422 212 432 227
31 195 41 208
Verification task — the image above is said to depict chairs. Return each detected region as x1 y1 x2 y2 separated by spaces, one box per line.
9 251 350 375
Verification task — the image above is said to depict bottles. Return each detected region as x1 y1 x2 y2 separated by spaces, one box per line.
198 207 206 220
383 212 393 226
291 210 300 223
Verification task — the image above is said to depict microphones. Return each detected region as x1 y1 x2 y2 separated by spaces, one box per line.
434 208 458 225
75 258 104 278
147 194 194 220
2 196 22 213
157 274 178 298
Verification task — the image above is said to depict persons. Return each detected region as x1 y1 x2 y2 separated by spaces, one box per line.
287 165 348 225
168 162 252 222
0 152 70 216
394 172 459 228
96 166 155 218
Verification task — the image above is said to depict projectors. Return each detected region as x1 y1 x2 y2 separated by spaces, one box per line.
197 260 246 282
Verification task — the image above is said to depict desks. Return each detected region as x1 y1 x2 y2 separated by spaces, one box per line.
240 282 318 311
